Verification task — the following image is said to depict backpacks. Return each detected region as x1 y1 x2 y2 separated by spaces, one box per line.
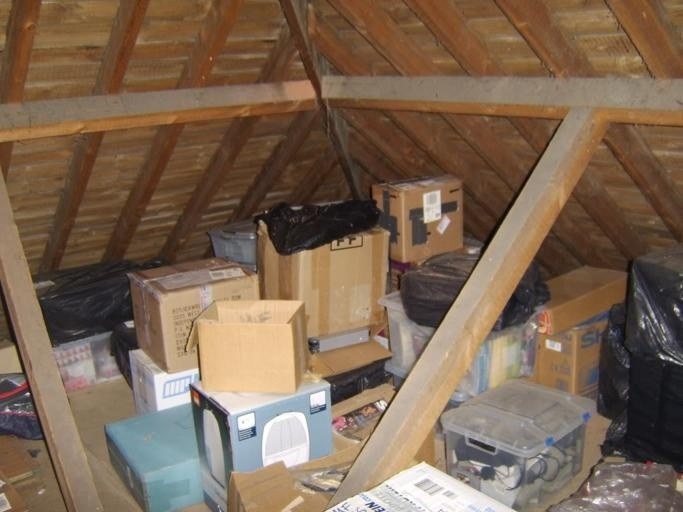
402 239 548 331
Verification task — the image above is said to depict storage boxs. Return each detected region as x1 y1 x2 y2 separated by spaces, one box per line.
32 171 639 511
